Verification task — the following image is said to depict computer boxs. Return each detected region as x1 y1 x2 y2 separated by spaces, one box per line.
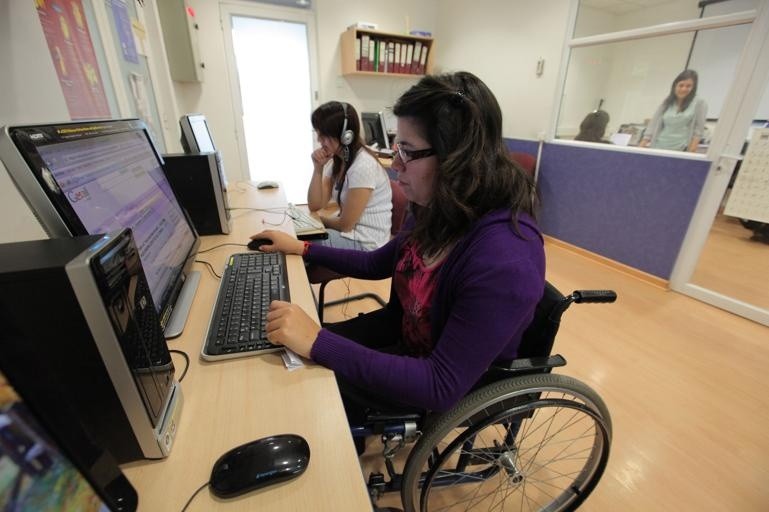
0 229 183 464
159 152 232 236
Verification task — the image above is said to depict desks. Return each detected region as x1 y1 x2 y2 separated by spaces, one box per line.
0 182 373 512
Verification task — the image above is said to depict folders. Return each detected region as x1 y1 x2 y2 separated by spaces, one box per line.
355 33 429 74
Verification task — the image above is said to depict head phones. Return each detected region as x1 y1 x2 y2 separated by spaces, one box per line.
338 102 353 145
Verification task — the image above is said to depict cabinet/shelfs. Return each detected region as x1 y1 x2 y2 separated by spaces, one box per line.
340 27 437 78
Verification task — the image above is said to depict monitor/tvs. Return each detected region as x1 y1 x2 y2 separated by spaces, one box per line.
0 331 136 511
178 113 218 154
0 117 202 340
360 111 391 150
614 123 646 146
745 119 769 142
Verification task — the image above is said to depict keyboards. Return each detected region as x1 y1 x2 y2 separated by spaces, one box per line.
290 202 326 234
202 251 292 361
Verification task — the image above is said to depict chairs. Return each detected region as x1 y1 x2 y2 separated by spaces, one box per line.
297 180 410 327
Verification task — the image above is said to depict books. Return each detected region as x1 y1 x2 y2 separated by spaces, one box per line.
356 35 432 75
347 22 377 30
412 31 431 37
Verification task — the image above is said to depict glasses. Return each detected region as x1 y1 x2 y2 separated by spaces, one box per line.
395 143 438 165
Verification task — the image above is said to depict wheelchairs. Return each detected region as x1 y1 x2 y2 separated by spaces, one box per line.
316 264 623 509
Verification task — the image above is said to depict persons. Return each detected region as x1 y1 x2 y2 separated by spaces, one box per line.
246 70 546 456
637 68 708 153
573 110 616 145
299 100 394 281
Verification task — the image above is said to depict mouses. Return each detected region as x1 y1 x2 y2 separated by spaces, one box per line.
247 238 273 249
258 181 278 189
209 432 310 498
